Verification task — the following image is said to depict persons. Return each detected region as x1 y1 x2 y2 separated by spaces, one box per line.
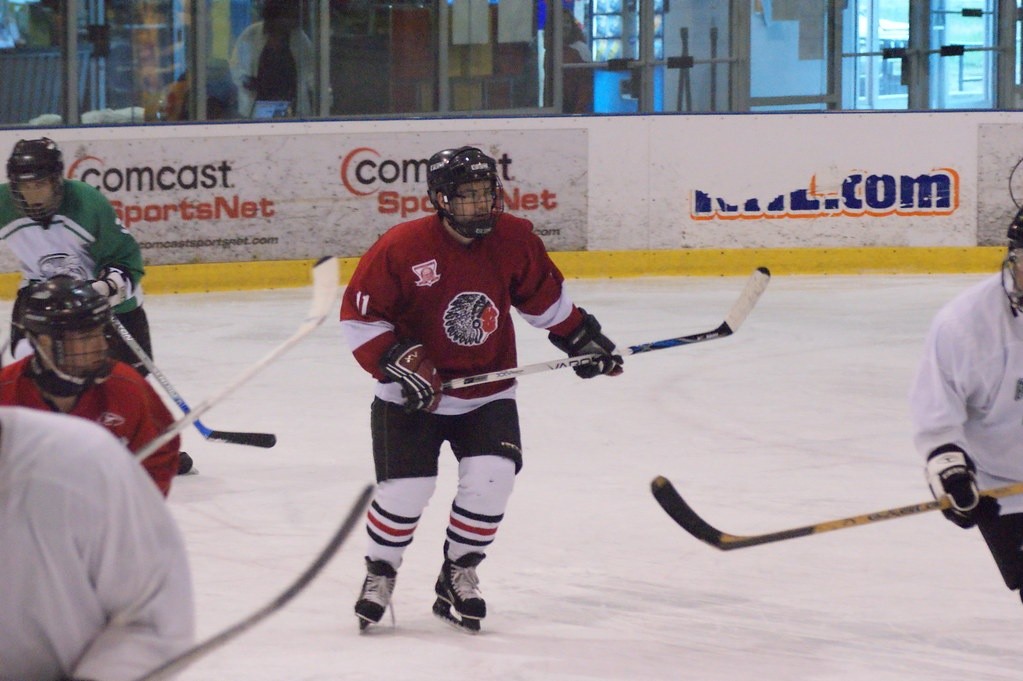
0 137 192 475
0 408 196 680
542 10 594 113
340 144 623 633
7 0 63 49
0 273 181 503
911 202 1023 606
230 0 317 119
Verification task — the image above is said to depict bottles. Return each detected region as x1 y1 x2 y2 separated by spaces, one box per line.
156 101 169 122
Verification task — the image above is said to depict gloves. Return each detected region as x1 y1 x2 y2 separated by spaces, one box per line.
548 307 624 379
378 339 443 414
924 445 1001 529
90 270 129 308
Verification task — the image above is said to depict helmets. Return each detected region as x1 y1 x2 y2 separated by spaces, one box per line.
426 146 504 239
1002 206 1023 314
11 275 120 396
7 136 65 223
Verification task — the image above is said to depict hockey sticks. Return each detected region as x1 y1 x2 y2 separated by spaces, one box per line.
650 474 1023 551
128 251 343 468
399 265 772 399
107 317 278 448
142 483 376 681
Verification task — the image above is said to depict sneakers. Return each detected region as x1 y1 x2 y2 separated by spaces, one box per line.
433 541 487 633
354 556 397 631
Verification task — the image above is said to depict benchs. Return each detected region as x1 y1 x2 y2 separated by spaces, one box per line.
0 48 92 128
